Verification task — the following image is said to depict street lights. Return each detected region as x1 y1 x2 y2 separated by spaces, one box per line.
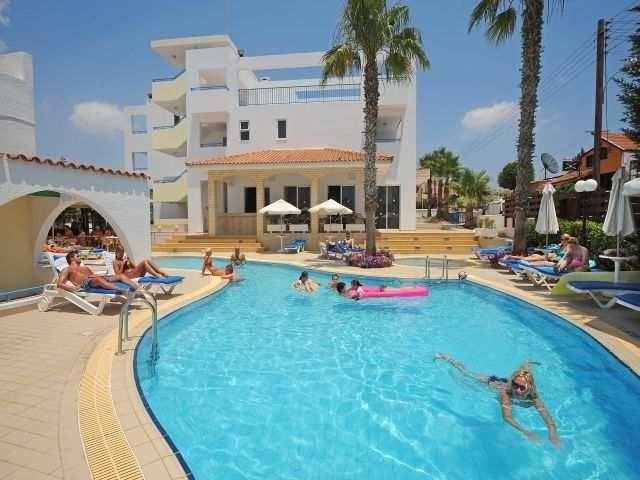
574 178 599 248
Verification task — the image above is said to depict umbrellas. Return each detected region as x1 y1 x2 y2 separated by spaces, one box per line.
306 199 353 235
602 161 637 256
260 199 301 235
534 181 561 250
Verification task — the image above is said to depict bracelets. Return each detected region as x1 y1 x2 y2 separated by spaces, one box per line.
546 422 556 427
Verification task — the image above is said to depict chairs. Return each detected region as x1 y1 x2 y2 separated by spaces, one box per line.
471 243 640 313
283 239 306 255
34 246 183 316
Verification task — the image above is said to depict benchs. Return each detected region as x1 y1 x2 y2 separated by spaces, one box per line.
323 223 365 233
266 223 309 234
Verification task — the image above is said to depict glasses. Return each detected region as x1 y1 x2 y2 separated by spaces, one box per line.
512 379 529 393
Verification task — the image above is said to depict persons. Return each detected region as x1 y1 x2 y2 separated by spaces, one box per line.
433 351 563 451
347 280 422 299
40 214 123 255
328 274 340 289
502 251 556 262
323 238 347 254
221 265 242 282
113 245 169 280
56 250 153 298
342 231 355 251
554 236 591 274
200 247 226 277
229 247 246 267
294 272 315 294
527 233 573 268
336 283 352 299
